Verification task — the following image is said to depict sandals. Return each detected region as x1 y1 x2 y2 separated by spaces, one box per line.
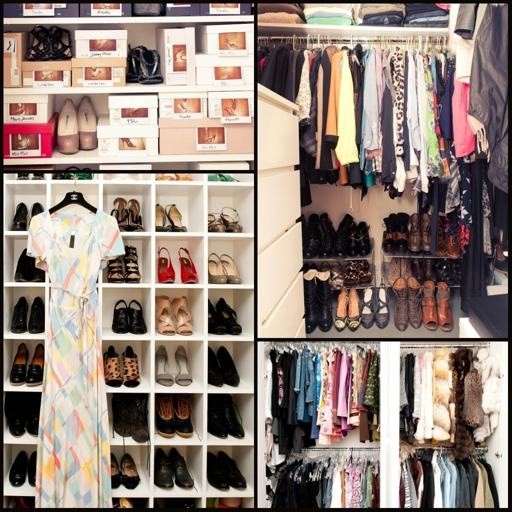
107 199 242 387
302 212 461 335
125 45 163 84
24 24 72 60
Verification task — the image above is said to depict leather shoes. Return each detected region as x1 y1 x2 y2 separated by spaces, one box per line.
111 395 248 491
57 95 98 154
4 200 46 487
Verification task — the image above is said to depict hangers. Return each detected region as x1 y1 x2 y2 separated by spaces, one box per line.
258 24 456 56
278 446 380 478
43 171 102 216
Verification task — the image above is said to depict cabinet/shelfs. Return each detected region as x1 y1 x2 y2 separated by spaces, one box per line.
255 83 307 341
3 170 256 508
